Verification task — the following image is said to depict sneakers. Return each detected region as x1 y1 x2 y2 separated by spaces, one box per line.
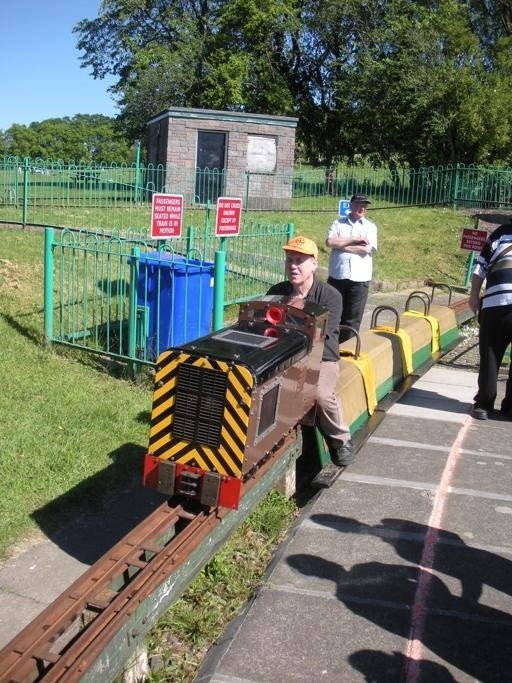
325 436 356 467
471 402 493 421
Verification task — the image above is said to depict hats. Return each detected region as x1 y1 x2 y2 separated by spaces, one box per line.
281 236 318 260
351 193 372 204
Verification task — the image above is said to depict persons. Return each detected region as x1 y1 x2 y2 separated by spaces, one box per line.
325 194 378 342
468 220 512 421
267 235 356 469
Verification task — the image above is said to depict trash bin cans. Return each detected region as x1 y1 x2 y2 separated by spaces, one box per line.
127 250 227 364
